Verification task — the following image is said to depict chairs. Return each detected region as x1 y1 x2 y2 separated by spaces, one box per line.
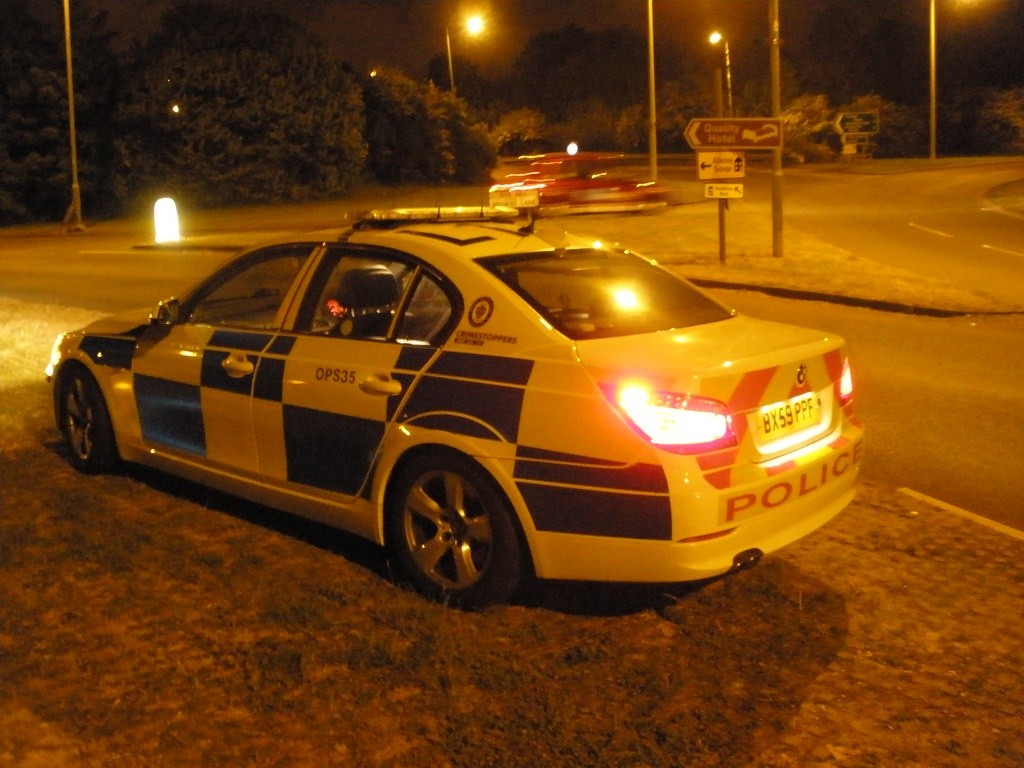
328 264 398 339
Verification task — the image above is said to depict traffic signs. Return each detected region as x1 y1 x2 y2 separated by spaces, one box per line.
697 151 745 181
834 110 879 135
682 117 784 151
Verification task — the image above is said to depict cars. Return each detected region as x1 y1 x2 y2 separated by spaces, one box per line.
45 205 868 610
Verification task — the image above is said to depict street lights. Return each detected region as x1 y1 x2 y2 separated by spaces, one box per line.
444 10 484 93
710 32 733 117
